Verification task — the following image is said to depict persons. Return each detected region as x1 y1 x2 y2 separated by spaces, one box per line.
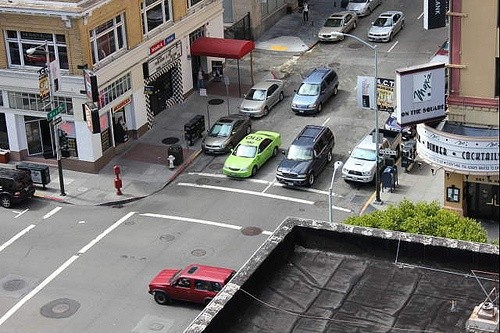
303 1 309 21
117 117 125 143
197 65 205 93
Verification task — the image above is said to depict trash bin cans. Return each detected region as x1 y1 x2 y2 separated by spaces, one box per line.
184 114 205 142
382 165 399 193
401 140 417 167
168 145 183 165
16 162 51 190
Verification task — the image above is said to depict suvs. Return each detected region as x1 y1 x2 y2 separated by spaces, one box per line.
290 67 339 114
147 264 237 308
275 125 336 188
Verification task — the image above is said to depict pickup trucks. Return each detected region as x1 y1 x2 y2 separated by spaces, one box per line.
341 128 402 185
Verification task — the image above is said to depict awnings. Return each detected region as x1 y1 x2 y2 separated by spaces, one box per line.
190 36 255 98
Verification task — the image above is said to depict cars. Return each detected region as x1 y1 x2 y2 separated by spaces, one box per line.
222 130 281 178
200 113 252 155
367 11 405 43
346 0 380 17
0 167 35 209
317 11 358 42
239 79 285 117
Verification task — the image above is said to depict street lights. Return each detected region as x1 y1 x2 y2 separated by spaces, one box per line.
26 39 67 197
331 32 383 206
